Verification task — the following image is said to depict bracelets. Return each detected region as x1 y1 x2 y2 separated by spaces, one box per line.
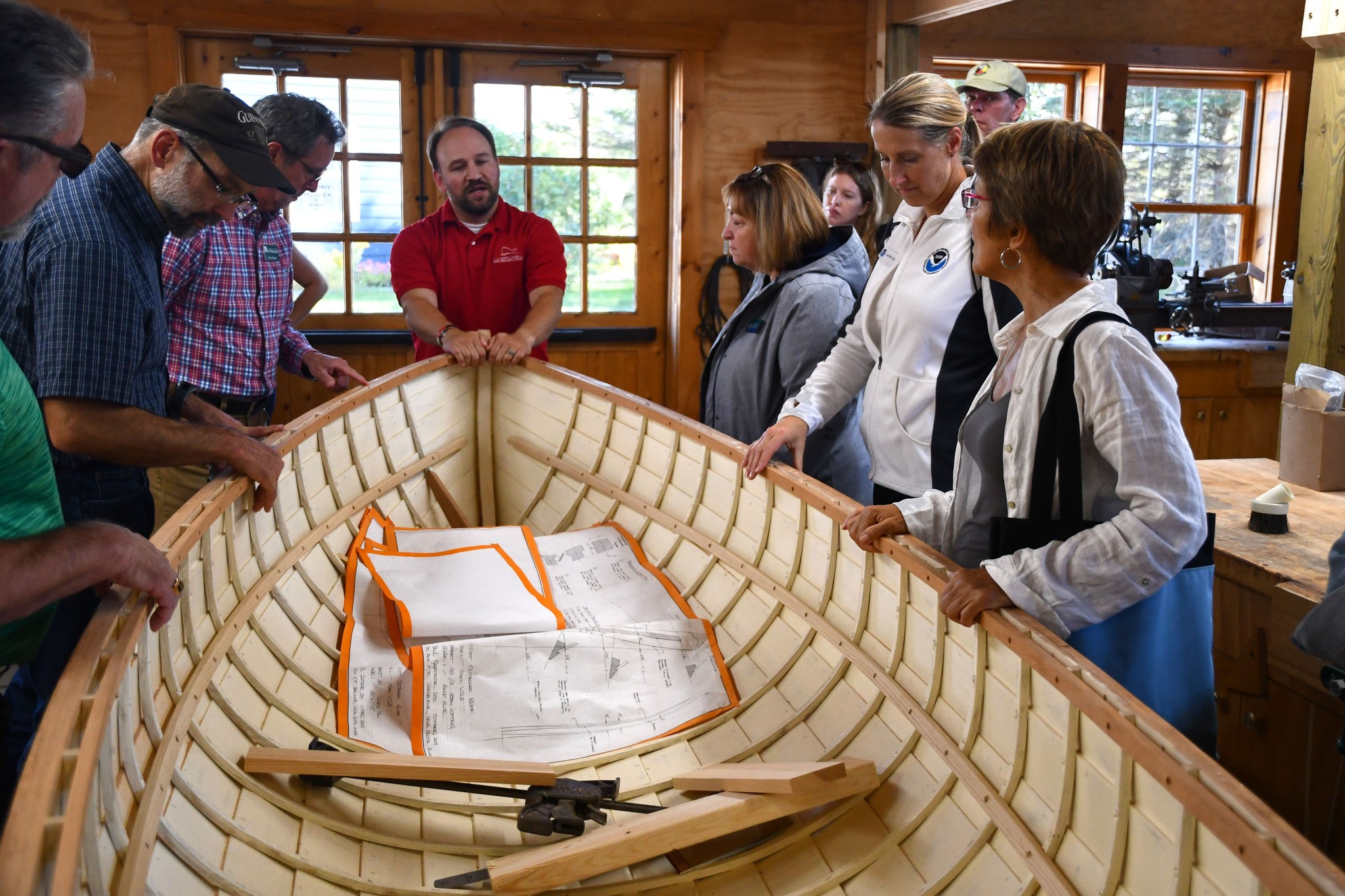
437 325 457 346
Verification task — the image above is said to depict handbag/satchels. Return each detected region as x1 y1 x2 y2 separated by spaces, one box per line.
991 511 1216 762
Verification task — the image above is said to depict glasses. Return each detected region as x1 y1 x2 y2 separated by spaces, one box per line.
282 145 329 182
178 135 260 208
750 165 772 185
2 132 93 180
961 188 994 210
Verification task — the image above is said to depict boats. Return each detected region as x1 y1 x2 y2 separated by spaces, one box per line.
0 344 1345 896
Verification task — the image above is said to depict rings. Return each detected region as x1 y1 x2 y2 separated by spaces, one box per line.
507 350 515 355
172 578 184 596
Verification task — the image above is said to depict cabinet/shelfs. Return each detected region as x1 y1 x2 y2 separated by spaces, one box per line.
1150 350 1286 462
1191 456 1345 875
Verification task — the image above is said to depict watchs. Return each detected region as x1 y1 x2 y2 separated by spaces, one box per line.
167 380 198 422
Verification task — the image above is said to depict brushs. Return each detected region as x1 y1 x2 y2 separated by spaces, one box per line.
1247 482 1294 534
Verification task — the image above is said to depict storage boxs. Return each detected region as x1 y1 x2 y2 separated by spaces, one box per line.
1277 382 1345 492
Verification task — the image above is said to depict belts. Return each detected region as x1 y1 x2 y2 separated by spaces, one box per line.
198 393 278 415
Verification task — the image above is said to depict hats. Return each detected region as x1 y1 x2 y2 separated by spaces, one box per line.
146 85 299 195
955 60 1031 101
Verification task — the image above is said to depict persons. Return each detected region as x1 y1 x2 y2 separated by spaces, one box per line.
697 162 884 511
390 117 567 369
1291 532 1345 866
159 92 370 484
0 0 300 841
740 57 1208 637
